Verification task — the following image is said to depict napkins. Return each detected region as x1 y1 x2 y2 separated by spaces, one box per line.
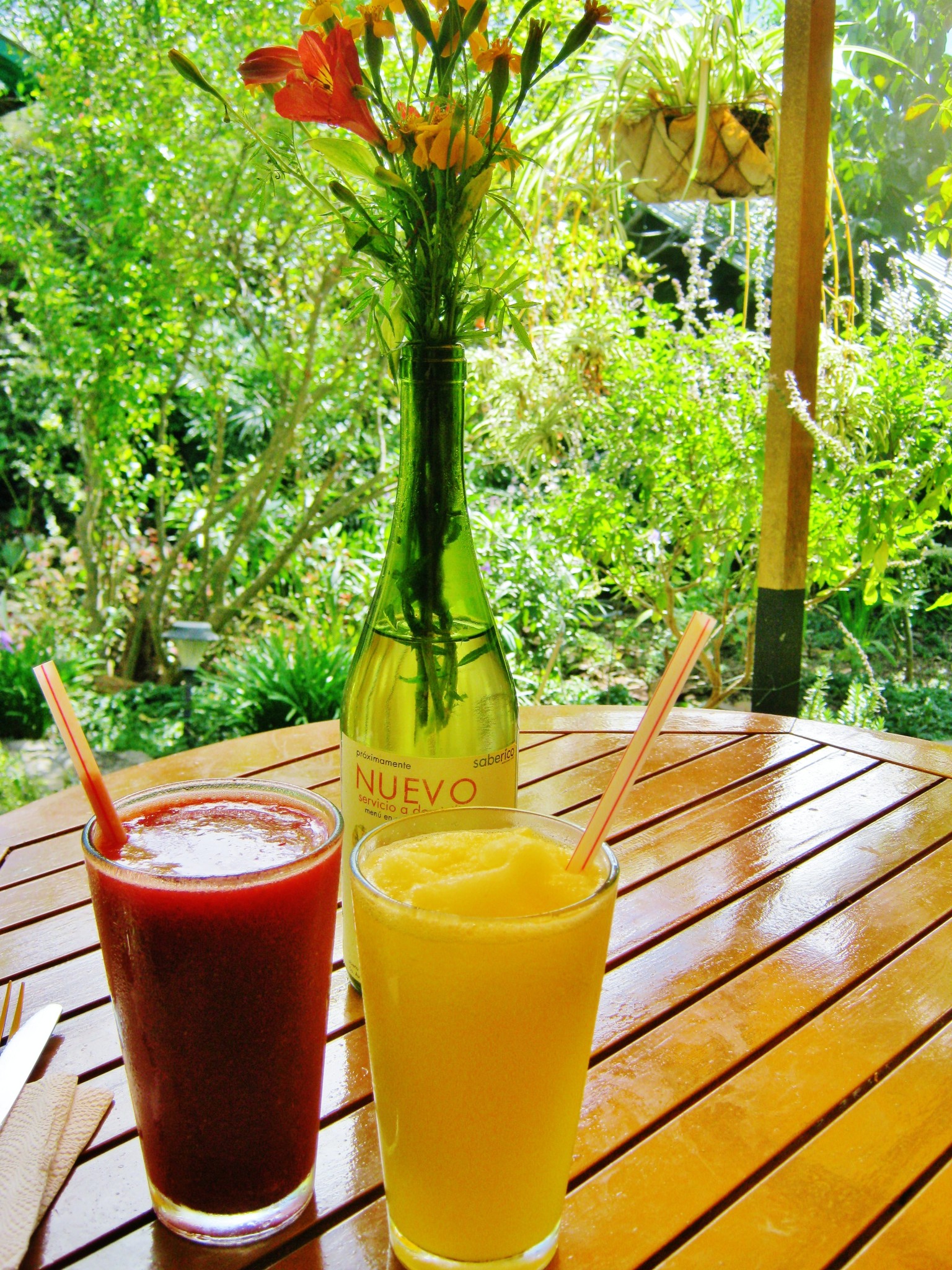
34 1086 115 1231
0 1073 78 1270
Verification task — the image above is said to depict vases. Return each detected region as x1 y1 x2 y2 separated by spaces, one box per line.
338 342 519 997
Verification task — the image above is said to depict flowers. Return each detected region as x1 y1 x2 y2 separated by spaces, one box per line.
170 0 616 744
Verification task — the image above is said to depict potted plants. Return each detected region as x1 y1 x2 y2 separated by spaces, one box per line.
516 0 926 343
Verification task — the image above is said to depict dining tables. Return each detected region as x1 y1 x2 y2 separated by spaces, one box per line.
0 707 952 1270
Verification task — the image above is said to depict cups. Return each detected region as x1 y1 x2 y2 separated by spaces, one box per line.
79 777 344 1247
351 803 619 1269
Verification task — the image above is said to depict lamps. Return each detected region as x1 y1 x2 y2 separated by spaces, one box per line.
161 620 220 749
